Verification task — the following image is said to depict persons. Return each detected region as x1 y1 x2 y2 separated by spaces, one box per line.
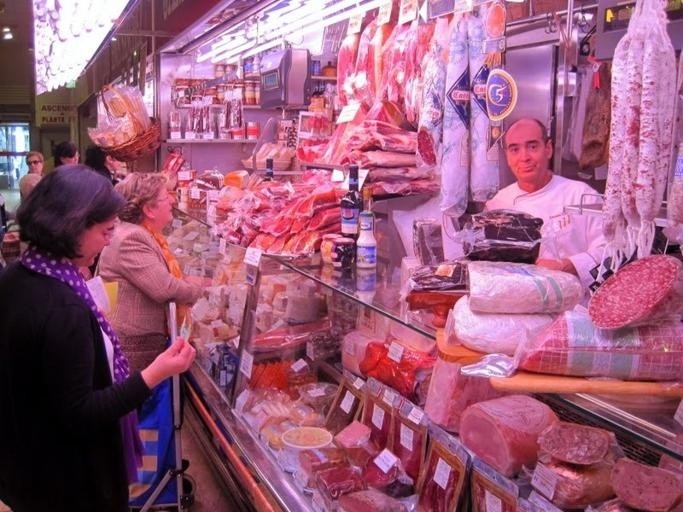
486 119 609 290
1 164 197 512
85 146 128 278
19 150 45 198
93 171 202 512
54 141 80 168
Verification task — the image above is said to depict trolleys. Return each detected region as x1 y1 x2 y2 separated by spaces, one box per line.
126 301 194 512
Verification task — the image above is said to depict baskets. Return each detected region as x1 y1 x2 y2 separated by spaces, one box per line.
241 159 290 170
99 86 160 162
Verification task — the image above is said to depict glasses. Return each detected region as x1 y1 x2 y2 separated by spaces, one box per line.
26 160 41 165
90 219 115 240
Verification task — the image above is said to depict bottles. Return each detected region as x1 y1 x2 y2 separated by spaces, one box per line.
174 50 269 105
321 234 342 264
306 60 337 105
357 211 377 268
340 165 364 240
331 238 355 271
358 187 377 234
263 157 274 182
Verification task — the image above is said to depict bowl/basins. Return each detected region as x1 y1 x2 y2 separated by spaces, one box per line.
281 427 333 468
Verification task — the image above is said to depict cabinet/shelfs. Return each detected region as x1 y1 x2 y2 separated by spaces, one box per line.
167 74 263 147
